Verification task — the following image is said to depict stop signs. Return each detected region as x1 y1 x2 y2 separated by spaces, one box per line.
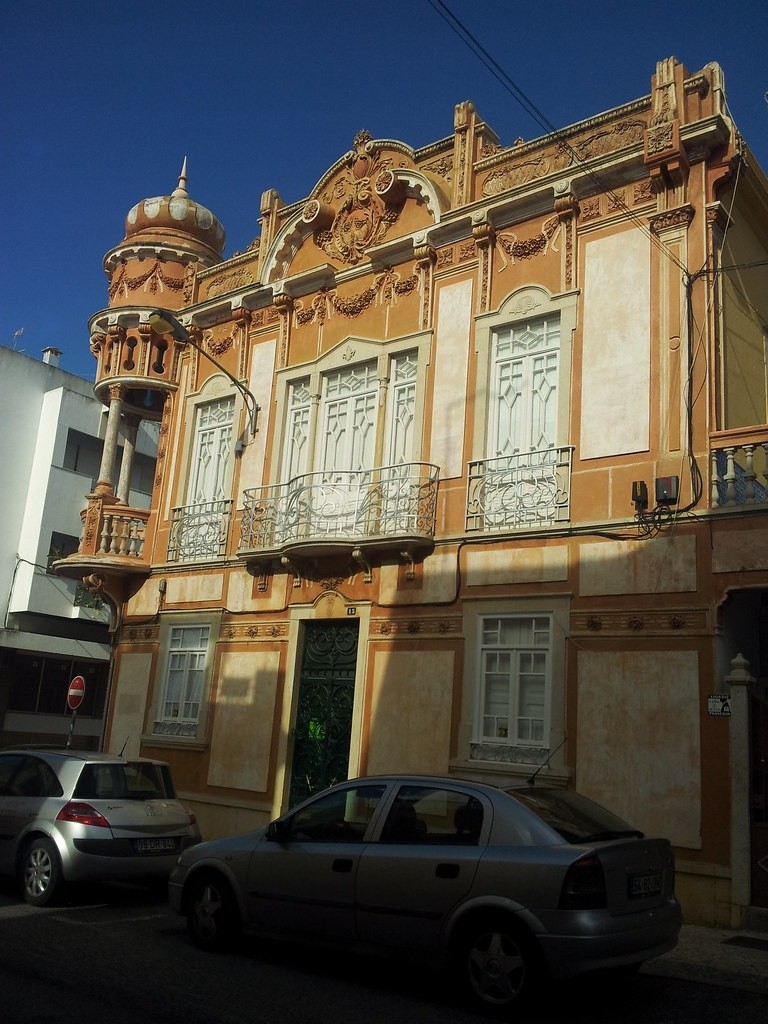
68 675 86 709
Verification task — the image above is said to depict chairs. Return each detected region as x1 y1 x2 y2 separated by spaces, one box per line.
436 803 483 846
382 804 427 838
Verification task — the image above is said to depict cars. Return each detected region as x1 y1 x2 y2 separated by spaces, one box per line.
166 774 684 1024
0 744 203 907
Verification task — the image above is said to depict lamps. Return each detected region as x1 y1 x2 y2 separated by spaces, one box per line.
148 311 258 435
